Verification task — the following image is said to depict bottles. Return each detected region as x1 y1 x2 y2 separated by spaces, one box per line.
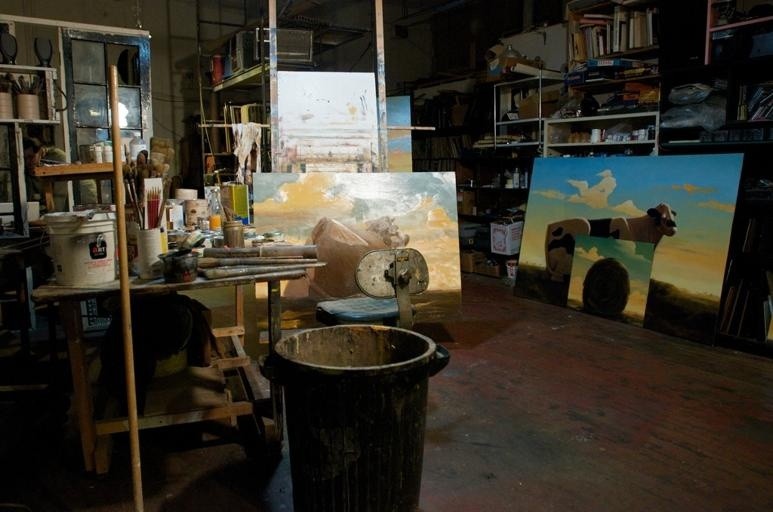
172 188 222 235
505 167 519 188
569 125 655 144
222 220 246 250
79 132 147 165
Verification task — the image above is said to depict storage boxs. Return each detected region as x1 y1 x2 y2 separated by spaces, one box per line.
491 223 522 255
459 249 505 277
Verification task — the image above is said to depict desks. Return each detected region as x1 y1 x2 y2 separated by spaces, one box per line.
29 232 304 472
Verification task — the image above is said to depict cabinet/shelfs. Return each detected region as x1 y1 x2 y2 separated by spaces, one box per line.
541 5 659 154
492 80 545 214
661 4 769 151
0 14 154 324
201 25 385 184
413 95 491 220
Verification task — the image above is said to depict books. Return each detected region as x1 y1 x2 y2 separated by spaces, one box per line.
572 5 656 63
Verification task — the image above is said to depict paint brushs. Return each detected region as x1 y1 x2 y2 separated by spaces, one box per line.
122 175 166 229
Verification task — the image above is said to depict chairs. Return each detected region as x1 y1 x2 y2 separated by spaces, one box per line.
313 248 428 332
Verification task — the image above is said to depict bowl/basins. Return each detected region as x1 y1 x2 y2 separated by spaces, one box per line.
158 252 202 283
203 173 238 186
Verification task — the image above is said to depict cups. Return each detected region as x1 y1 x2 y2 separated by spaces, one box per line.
1 92 41 119
137 229 164 280
520 171 528 188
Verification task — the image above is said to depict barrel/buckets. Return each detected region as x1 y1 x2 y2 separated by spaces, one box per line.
39 212 119 286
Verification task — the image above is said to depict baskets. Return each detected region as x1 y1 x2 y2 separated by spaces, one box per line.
449 92 472 128
475 259 507 278
